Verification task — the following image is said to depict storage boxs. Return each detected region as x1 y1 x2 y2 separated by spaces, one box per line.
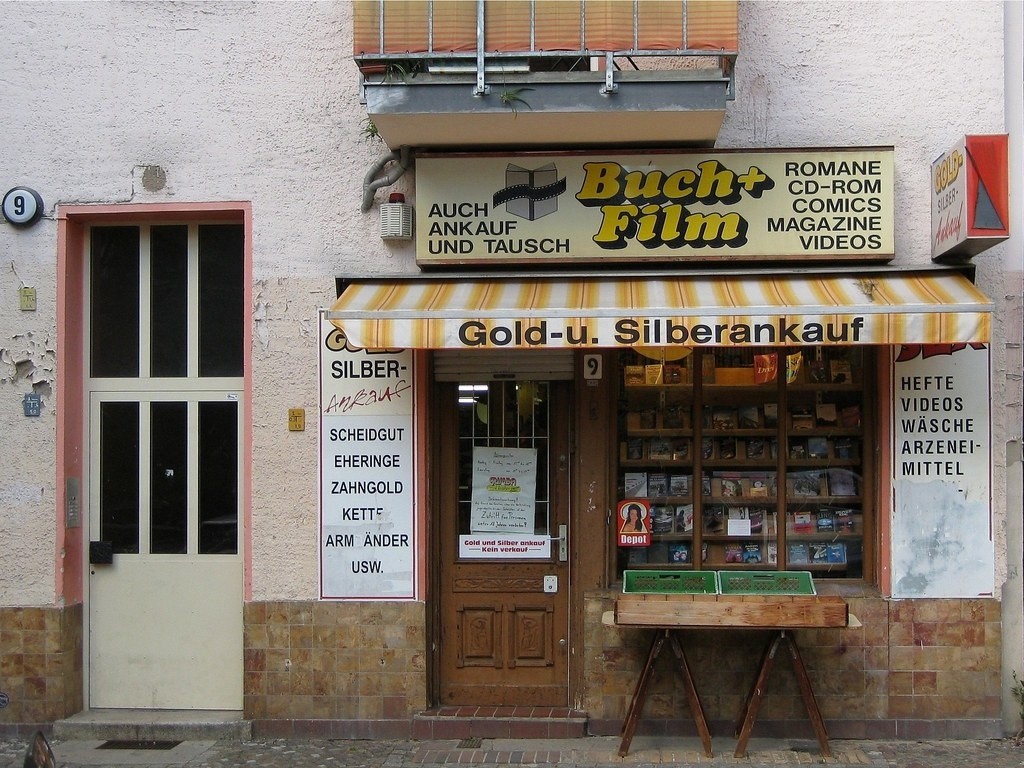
623 570 817 595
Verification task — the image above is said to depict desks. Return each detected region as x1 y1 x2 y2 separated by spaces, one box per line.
602 610 863 758
